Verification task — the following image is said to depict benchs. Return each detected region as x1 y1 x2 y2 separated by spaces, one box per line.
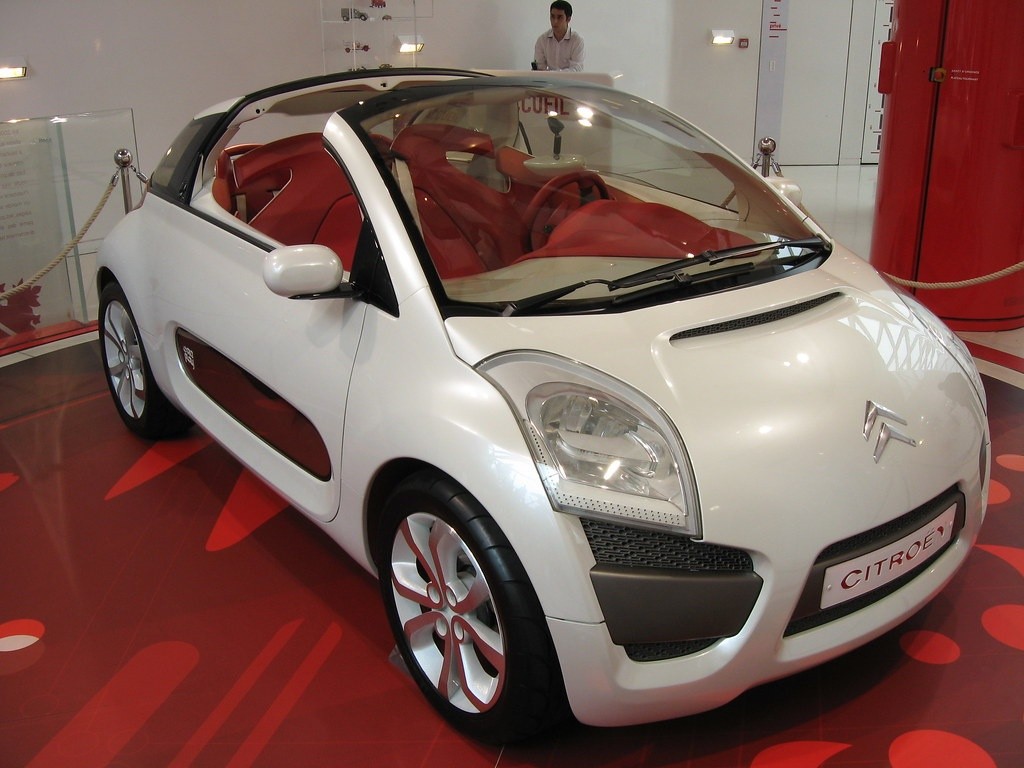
212 123 644 281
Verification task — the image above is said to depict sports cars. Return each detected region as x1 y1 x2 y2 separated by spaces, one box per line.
92 66 995 743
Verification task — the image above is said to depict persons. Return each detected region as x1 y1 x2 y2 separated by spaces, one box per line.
534 0 584 73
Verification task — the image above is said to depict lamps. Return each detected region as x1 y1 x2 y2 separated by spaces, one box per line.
392 34 425 55
707 29 735 46
0 55 32 81
